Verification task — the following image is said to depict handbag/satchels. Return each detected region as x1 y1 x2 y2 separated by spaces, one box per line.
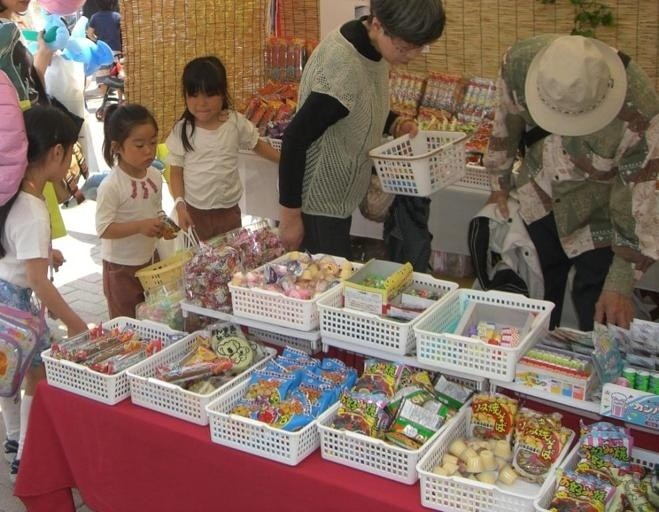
0 304 43 396
51 140 89 203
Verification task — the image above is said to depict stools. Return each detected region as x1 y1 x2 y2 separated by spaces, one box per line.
98 73 127 115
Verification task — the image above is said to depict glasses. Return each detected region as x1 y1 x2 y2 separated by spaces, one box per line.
390 36 429 56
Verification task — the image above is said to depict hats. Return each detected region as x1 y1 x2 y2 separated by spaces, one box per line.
525 34 626 137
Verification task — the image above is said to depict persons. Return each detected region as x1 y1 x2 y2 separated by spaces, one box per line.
87 1 124 120
1 106 78 476
96 103 178 320
0 1 53 108
276 2 445 258
165 56 279 247
470 35 651 335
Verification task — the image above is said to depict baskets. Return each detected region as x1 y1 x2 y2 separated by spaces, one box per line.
136 248 192 288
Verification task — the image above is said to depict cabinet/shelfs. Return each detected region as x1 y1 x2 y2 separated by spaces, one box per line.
12 299 659 512
242 144 492 260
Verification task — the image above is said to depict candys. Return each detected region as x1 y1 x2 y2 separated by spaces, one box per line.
184 245 240 311
224 227 283 272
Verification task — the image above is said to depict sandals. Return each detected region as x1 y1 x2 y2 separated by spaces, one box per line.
5 441 19 463
10 458 21 484
